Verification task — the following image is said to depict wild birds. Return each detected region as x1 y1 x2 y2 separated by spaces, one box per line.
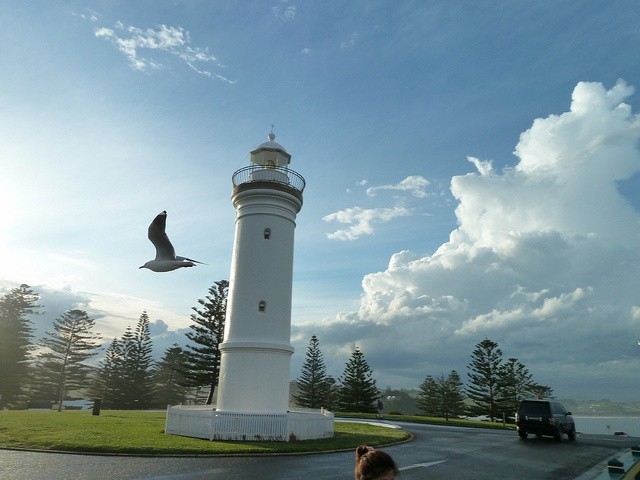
138 210 210 273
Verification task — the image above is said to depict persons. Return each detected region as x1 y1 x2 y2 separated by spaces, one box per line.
354 445 397 480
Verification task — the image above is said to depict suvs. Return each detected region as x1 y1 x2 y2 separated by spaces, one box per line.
515 399 575 442
